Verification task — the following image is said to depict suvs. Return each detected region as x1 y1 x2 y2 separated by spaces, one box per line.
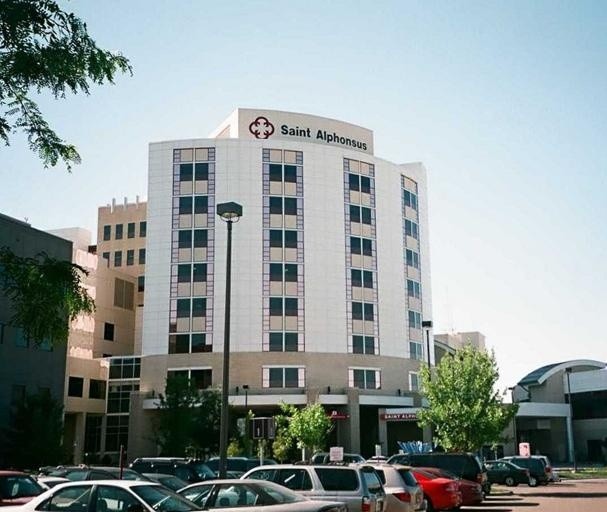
131 457 218 481
503 456 547 487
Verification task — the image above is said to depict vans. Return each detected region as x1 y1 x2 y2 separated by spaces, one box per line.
209 457 278 479
504 456 553 485
239 465 388 512
385 452 488 484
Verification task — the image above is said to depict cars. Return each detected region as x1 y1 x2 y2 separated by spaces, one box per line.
373 465 427 512
144 473 188 491
0 471 50 505
58 467 148 481
43 476 69 488
484 461 529 486
0 479 203 512
152 479 349 512
412 468 463 512
419 466 482 503
312 453 388 466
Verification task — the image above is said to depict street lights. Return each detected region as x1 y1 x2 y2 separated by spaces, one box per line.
218 203 240 471
